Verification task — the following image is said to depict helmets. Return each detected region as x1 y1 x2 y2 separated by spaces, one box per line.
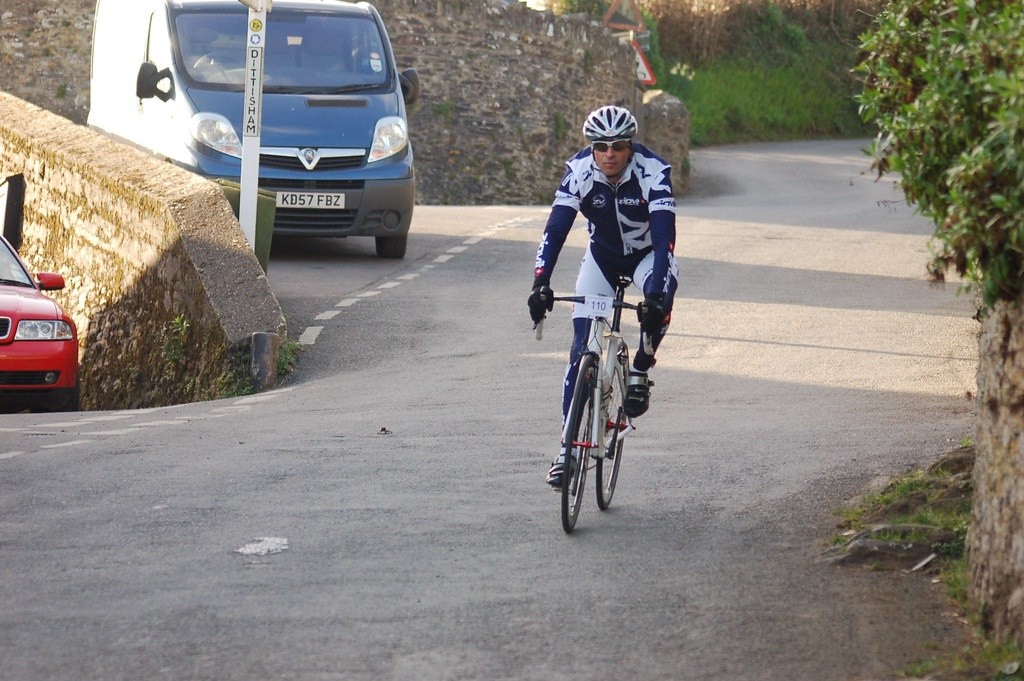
583 106 639 141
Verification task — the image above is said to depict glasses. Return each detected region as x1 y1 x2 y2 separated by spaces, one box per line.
592 140 632 152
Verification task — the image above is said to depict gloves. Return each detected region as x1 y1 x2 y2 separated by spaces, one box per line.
528 286 554 324
640 293 665 333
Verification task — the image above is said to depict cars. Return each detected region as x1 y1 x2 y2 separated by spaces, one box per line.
0 235 83 414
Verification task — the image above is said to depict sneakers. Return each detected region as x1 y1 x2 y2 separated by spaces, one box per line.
545 454 577 486
624 371 654 417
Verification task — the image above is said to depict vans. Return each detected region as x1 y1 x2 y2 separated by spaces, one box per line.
86 0 421 261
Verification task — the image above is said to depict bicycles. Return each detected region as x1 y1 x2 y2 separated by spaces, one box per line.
531 270 655 535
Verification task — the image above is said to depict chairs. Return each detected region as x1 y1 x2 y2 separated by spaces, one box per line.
182 20 219 63
299 27 349 71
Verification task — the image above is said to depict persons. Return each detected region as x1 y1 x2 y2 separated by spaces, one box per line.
528 105 679 485
